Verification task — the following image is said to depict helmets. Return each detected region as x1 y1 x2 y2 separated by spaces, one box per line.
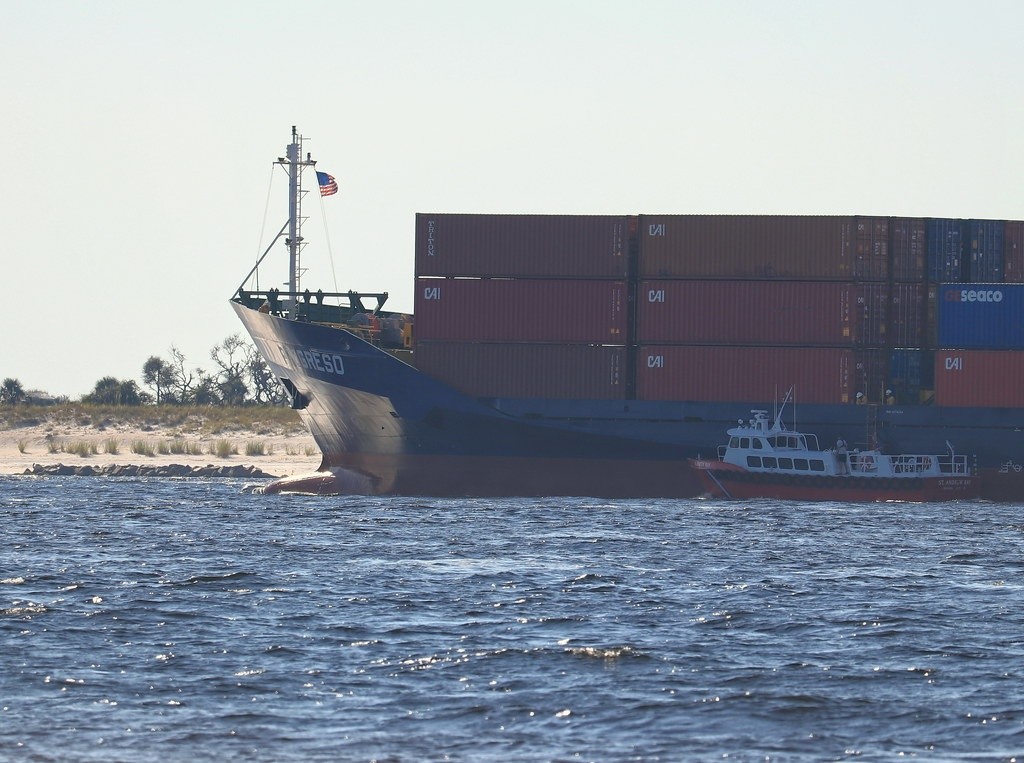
885 389 892 395
856 392 863 399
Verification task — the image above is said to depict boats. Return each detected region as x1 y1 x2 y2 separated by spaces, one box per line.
686 383 981 500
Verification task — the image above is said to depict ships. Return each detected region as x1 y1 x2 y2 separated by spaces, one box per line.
228 121 1023 499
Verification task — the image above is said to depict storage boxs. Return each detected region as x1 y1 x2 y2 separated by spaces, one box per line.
415 213 1023 408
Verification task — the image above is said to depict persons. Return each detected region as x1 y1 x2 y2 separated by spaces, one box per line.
905 458 914 470
923 456 929 470
895 457 902 473
885 389 896 406
829 436 851 475
870 435 883 450
855 391 867 405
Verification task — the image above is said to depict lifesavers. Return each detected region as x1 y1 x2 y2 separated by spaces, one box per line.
714 469 932 490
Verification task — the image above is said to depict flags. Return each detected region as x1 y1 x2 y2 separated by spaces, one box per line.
316 171 339 197
